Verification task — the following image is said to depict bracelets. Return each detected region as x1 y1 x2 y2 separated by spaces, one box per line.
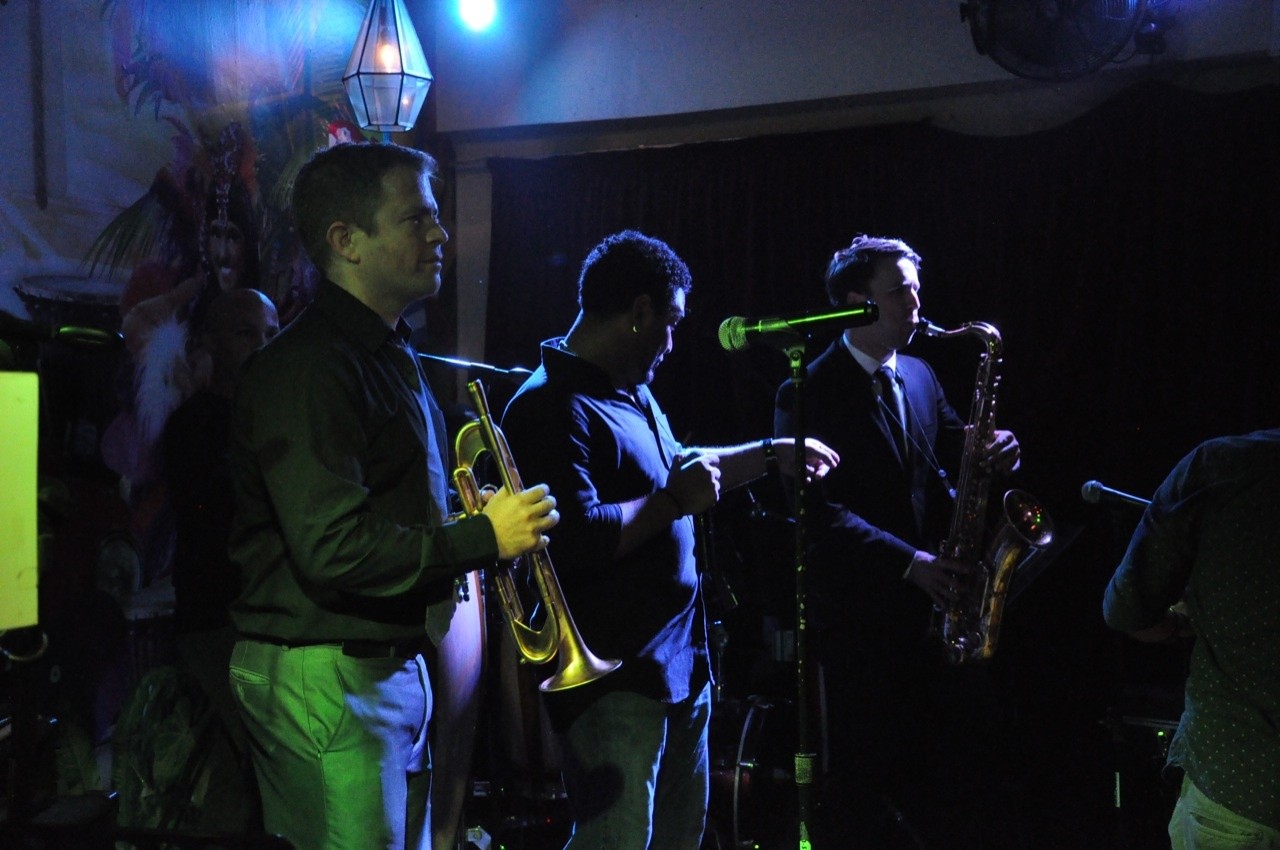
656 487 683 520
764 439 780 481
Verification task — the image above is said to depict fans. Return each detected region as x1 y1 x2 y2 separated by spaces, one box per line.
958 0 1169 82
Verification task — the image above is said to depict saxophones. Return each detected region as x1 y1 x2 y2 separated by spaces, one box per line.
918 312 1057 657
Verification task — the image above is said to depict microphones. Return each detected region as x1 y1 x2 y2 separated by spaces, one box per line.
1081 480 1152 513
684 451 717 571
718 301 880 353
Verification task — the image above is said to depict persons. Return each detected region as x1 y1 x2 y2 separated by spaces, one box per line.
1104 431 1280 850
496 231 840 850
100 196 279 850
774 234 1021 850
230 143 560 850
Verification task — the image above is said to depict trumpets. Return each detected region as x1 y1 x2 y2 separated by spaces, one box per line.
449 378 625 693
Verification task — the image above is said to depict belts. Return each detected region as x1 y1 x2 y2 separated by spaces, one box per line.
342 639 390 658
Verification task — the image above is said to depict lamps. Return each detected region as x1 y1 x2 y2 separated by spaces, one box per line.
342 0 435 133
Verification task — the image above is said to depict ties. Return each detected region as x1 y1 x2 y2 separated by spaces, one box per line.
875 365 910 462
395 338 455 648
